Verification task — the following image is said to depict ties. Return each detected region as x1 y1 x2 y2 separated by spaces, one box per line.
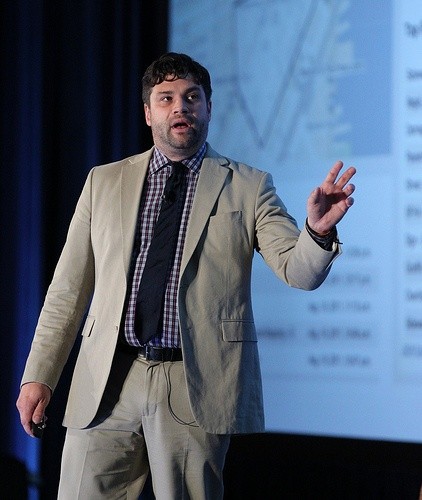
134 162 189 345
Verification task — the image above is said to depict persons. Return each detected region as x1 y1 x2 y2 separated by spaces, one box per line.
15 52 356 500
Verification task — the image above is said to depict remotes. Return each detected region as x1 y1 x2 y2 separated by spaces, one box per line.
30 416 45 438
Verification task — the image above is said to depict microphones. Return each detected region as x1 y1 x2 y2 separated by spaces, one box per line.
165 191 177 201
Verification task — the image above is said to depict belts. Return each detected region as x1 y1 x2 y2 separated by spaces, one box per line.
117 345 183 363
